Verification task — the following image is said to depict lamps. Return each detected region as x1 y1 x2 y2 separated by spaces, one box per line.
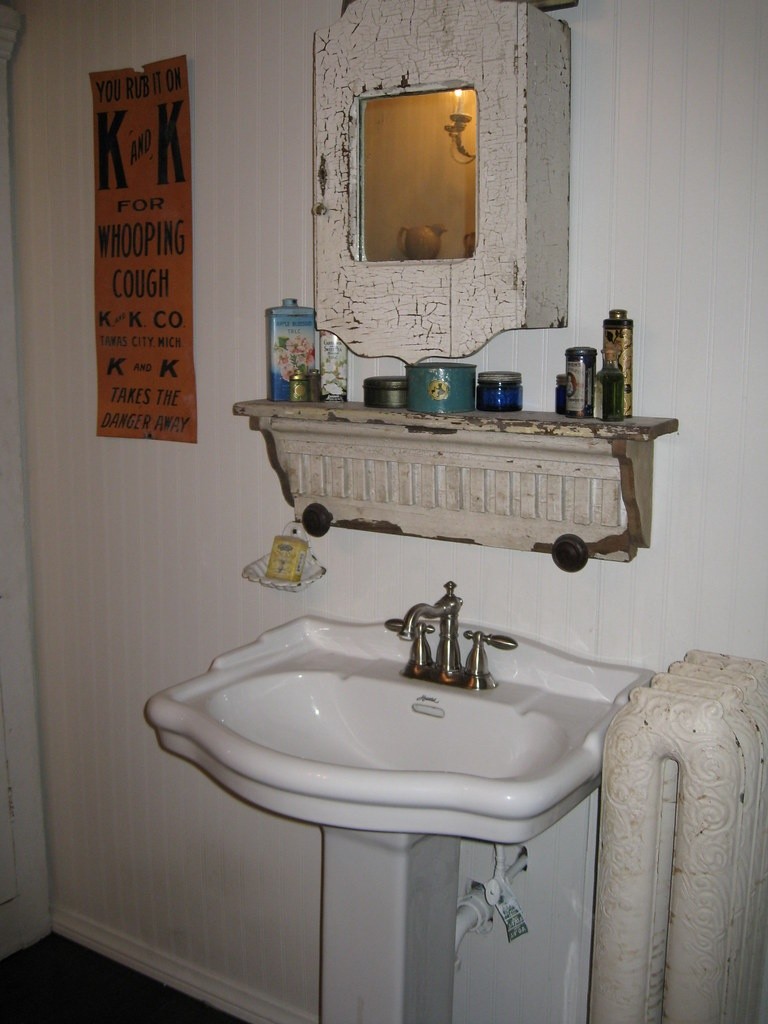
442 87 476 166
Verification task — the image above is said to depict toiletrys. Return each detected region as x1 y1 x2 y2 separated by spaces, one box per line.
592 347 625 422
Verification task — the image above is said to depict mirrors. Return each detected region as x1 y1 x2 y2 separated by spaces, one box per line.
348 79 483 265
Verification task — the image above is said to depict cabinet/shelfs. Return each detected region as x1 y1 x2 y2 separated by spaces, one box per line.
313 0 576 367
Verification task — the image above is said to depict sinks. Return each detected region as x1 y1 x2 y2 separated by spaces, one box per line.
143 613 658 846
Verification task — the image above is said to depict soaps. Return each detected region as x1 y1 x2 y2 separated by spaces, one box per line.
264 534 309 583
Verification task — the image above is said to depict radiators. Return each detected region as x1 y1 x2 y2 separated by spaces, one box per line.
582 650 768 1024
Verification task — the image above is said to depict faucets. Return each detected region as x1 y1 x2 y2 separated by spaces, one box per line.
398 580 463 687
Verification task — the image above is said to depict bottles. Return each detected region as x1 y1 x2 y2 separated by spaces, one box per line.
565 347 597 420
477 371 523 412
290 369 320 402
266 299 315 402
603 309 634 418
555 374 568 415
593 350 624 422
